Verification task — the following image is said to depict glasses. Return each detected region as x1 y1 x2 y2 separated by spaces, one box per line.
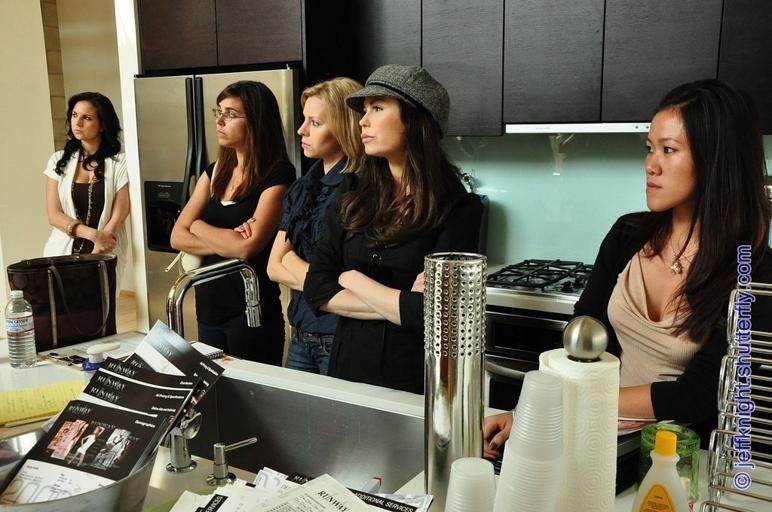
213 108 247 122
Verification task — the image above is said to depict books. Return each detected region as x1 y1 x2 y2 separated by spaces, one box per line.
0 319 225 505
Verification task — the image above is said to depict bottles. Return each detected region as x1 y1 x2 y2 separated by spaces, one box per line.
5 286 38 368
628 432 688 510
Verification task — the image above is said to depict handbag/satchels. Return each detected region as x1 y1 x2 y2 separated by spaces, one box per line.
164 250 202 272
8 252 116 352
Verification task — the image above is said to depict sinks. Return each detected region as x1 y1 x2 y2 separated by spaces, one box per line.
161 374 425 493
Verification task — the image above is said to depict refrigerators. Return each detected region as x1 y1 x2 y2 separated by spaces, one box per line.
131 62 307 368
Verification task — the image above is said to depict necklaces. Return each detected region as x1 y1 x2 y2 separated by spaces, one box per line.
668 233 699 273
68 162 98 253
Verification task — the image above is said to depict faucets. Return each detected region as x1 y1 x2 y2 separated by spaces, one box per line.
166 258 262 473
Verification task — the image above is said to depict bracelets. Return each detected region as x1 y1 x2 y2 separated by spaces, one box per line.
511 409 516 424
67 219 82 238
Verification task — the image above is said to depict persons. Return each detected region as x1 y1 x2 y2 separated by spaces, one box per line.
482 82 772 460
265 77 365 376
170 81 295 367
302 65 484 395
43 92 130 336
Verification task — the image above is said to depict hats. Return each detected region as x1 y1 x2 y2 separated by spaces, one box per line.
345 64 450 139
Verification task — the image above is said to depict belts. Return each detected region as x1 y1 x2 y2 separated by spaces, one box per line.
288 326 334 348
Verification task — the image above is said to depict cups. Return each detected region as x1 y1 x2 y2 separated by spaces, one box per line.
446 456 495 512
493 367 570 512
638 417 702 506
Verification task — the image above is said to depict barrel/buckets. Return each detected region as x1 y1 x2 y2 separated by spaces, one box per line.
0 430 159 512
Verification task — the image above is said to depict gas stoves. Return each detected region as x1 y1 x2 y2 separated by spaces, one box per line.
484 256 597 316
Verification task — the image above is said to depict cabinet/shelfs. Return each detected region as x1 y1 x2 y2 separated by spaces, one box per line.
502 0 772 136
359 0 504 137
484 309 570 411
136 0 348 75
